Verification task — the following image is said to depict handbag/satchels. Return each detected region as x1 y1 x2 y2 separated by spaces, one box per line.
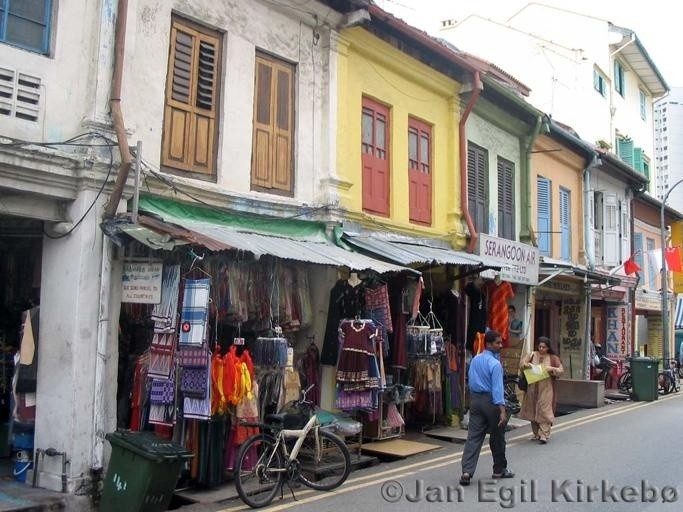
519 367 527 390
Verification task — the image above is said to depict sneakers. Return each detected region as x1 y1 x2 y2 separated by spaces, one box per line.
532 435 547 443
492 469 514 478
459 472 471 486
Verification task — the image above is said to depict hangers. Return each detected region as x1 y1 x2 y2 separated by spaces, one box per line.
181 256 211 280
351 314 362 327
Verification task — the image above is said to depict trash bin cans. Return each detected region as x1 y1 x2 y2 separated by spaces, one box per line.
99 432 196 512
626 355 660 401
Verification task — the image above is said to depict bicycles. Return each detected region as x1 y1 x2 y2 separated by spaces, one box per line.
501 363 527 415
618 358 681 395
235 384 350 507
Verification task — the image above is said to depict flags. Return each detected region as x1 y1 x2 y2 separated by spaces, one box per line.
663 246 682 272
622 253 642 275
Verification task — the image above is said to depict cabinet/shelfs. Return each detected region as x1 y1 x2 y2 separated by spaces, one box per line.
361 390 405 441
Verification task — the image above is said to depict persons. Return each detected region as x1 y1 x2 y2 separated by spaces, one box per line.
506 305 522 338
518 335 564 444
459 329 516 485
